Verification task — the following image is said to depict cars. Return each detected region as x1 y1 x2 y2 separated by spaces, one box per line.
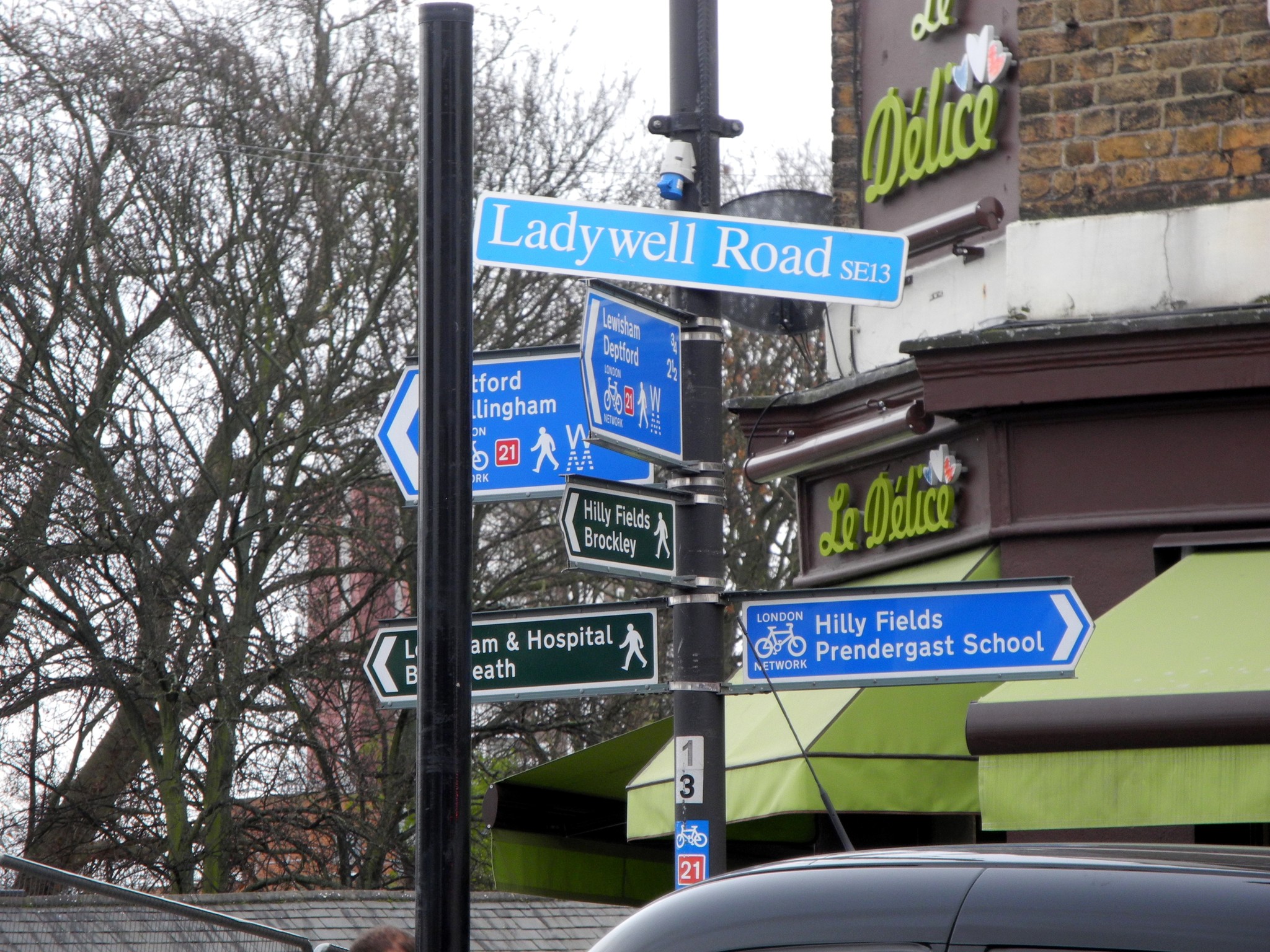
582 843 1269 952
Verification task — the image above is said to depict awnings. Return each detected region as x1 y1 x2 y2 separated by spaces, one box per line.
483 715 819 907
626 549 1000 842
965 553 1270 832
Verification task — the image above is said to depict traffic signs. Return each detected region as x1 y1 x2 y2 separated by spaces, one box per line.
580 275 689 463
372 350 656 508
724 572 1094 691
476 189 908 309
363 597 668 712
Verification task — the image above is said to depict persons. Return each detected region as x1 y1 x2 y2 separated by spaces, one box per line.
350 925 416 952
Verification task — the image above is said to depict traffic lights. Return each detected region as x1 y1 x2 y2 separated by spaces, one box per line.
557 473 685 582
